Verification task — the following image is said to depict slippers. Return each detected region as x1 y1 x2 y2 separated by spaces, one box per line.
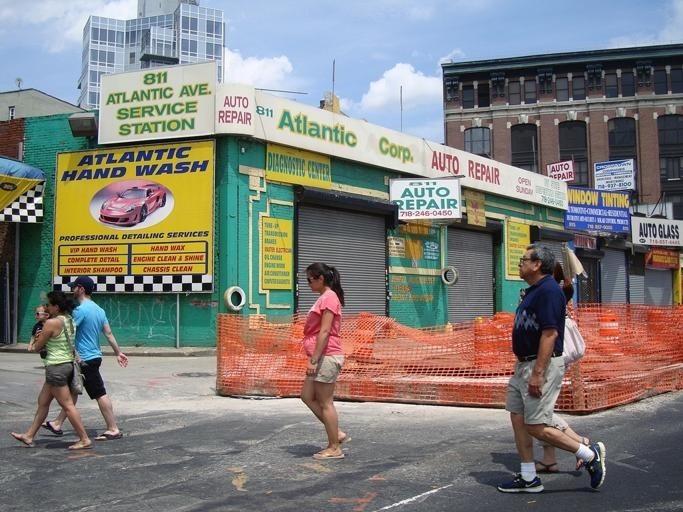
8 421 63 448
67 431 123 450
312 432 352 459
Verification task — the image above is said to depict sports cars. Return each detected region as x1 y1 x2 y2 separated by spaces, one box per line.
99 184 165 227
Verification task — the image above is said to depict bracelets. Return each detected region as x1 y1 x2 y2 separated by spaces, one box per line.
310 360 319 365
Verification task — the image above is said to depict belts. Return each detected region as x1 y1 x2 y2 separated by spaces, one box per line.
518 353 561 361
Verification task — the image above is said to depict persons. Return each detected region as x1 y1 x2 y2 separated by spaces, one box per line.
300 262 353 460
496 243 606 493
41 275 128 442
26 302 50 359
520 260 592 474
9 289 94 451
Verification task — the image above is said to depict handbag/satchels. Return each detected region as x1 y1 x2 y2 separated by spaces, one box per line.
70 359 83 396
564 323 585 362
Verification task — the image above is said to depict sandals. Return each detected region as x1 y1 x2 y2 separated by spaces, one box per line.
535 437 591 473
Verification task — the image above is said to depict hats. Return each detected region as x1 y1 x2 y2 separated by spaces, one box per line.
68 276 94 294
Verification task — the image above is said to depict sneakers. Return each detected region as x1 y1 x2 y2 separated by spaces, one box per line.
583 442 607 490
497 473 544 493
